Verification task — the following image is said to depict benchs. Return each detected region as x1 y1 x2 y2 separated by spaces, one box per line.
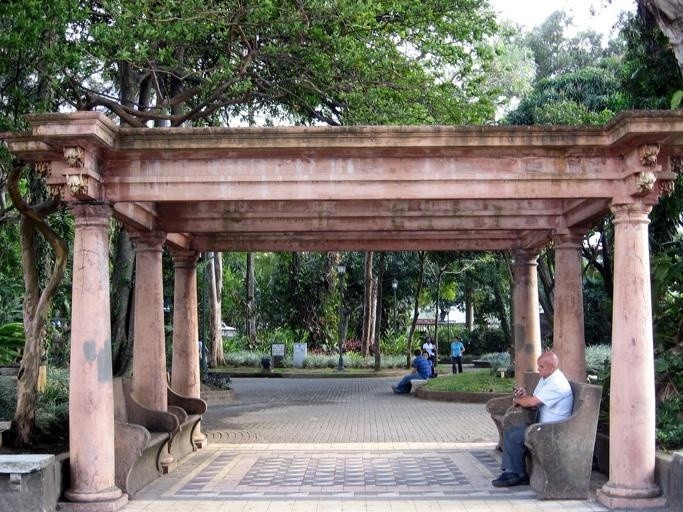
161 369 207 474
502 372 603 500
410 377 430 393
485 370 540 450
113 377 179 500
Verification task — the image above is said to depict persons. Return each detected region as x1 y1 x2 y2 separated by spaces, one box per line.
449 334 466 375
421 335 436 363
197 336 209 368
391 348 431 395
490 350 575 487
401 351 435 393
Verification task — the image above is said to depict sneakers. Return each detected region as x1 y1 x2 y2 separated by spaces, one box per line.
492 471 529 488
